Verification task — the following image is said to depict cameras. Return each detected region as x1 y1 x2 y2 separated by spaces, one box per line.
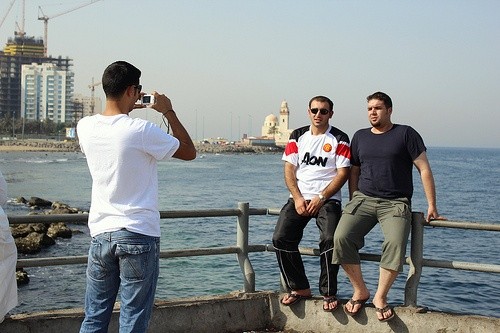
141 95 155 105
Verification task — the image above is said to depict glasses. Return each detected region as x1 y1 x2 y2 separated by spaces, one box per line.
134 85 142 91
310 108 331 115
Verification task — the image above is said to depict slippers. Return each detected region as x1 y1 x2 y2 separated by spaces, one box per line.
280 292 312 306
344 295 369 316
323 296 338 312
375 303 395 322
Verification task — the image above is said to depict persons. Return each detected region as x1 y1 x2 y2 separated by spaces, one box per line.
331 92 447 321
77 61 196 333
273 96 352 312
0 172 17 323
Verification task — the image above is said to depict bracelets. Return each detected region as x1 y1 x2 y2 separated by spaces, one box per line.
319 195 326 202
163 110 174 115
